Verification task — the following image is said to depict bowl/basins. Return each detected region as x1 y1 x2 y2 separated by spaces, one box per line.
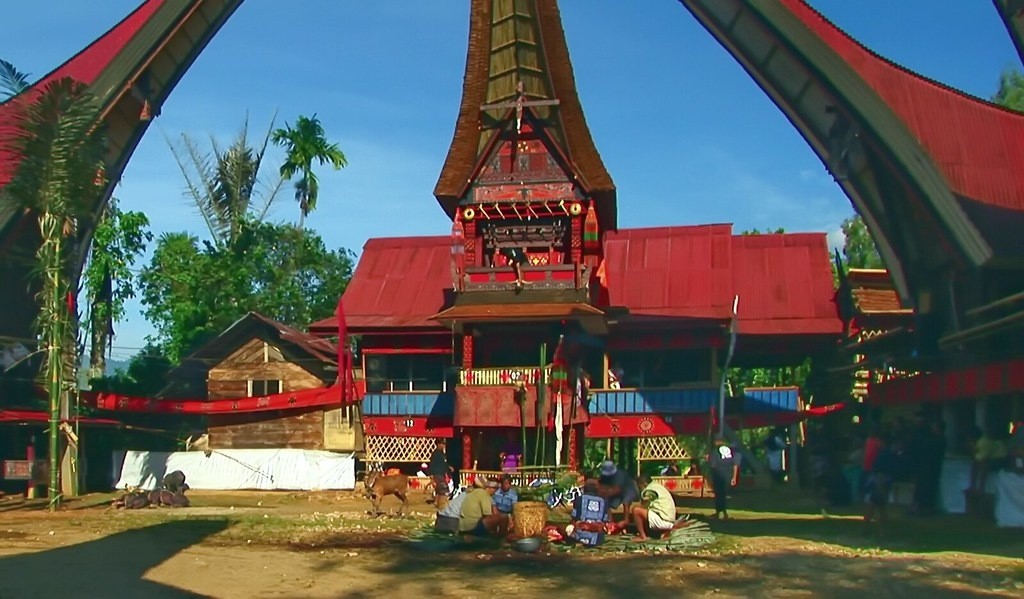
516 537 541 552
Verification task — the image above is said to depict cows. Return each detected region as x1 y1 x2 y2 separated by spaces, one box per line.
357 469 408 514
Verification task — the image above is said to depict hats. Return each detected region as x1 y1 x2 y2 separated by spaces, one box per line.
601 460 617 476
474 477 489 489
434 436 446 445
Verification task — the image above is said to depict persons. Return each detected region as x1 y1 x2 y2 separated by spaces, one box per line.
687 459 702 476
630 474 676 542
595 461 640 528
491 472 518 533
705 434 740 521
960 422 1009 533
662 459 681 476
428 438 454 509
861 410 947 516
457 476 509 537
